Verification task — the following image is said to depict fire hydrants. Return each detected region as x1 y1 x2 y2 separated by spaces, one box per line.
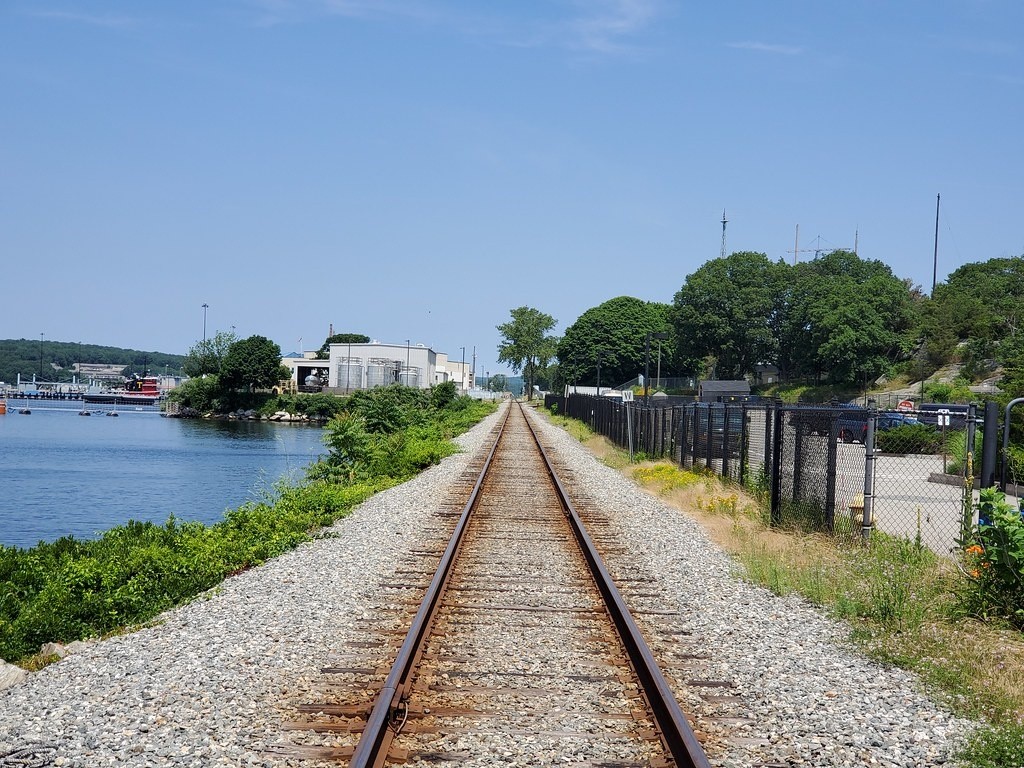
851 494 880 534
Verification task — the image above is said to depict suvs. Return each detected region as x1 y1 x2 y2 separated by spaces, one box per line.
789 398 862 436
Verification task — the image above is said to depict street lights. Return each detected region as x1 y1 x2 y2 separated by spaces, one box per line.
232 325 236 334
40 332 46 381
653 333 669 387
201 303 209 353
347 334 353 393
460 347 465 390
79 342 81 382
405 339 410 387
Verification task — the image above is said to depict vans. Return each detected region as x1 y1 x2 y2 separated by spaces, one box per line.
676 402 751 457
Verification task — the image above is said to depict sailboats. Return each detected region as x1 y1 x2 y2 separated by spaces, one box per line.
106 399 118 417
19 396 31 415
78 399 90 416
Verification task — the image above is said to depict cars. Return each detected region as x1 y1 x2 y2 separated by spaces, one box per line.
833 409 925 446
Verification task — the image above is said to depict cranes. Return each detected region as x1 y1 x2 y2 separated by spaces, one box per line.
785 236 851 259
